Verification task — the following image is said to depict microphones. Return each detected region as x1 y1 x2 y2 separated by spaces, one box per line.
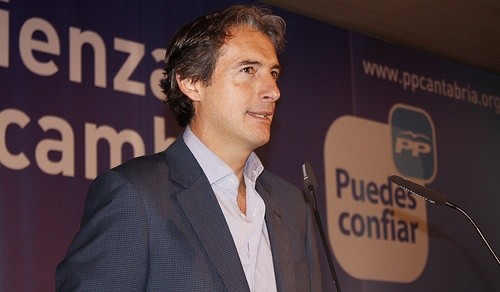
391 175 500 266
301 161 341 292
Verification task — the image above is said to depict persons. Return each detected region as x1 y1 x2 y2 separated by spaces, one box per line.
56 5 322 292
